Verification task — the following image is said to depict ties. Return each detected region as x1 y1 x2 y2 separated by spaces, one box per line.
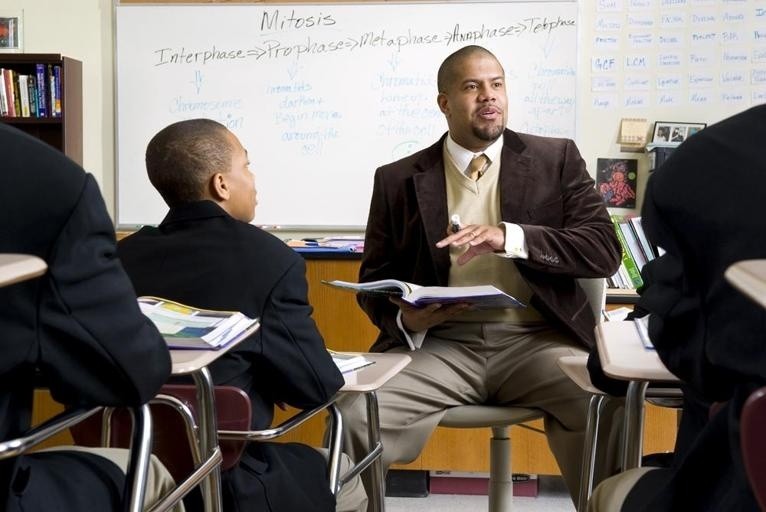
469 154 488 181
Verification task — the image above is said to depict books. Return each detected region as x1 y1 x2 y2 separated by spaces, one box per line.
130 284 261 355
318 339 374 377
632 303 665 353
0 58 63 116
601 210 692 288
317 268 530 317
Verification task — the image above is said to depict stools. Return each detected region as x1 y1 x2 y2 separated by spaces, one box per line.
438 404 544 512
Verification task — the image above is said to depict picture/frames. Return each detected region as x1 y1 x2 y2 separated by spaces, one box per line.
651 120 706 146
590 152 649 218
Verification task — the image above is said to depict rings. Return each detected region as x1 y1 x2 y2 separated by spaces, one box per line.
468 231 481 240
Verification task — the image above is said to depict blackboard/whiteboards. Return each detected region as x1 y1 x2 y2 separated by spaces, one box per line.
112 0 765 232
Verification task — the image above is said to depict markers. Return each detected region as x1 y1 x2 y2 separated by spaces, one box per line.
449 214 461 234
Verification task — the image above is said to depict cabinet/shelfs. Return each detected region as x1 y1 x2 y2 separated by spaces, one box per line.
0 53 82 167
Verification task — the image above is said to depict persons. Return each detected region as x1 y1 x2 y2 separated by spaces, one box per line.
114 116 377 511
318 44 626 512
580 98 766 510
1 120 194 512
